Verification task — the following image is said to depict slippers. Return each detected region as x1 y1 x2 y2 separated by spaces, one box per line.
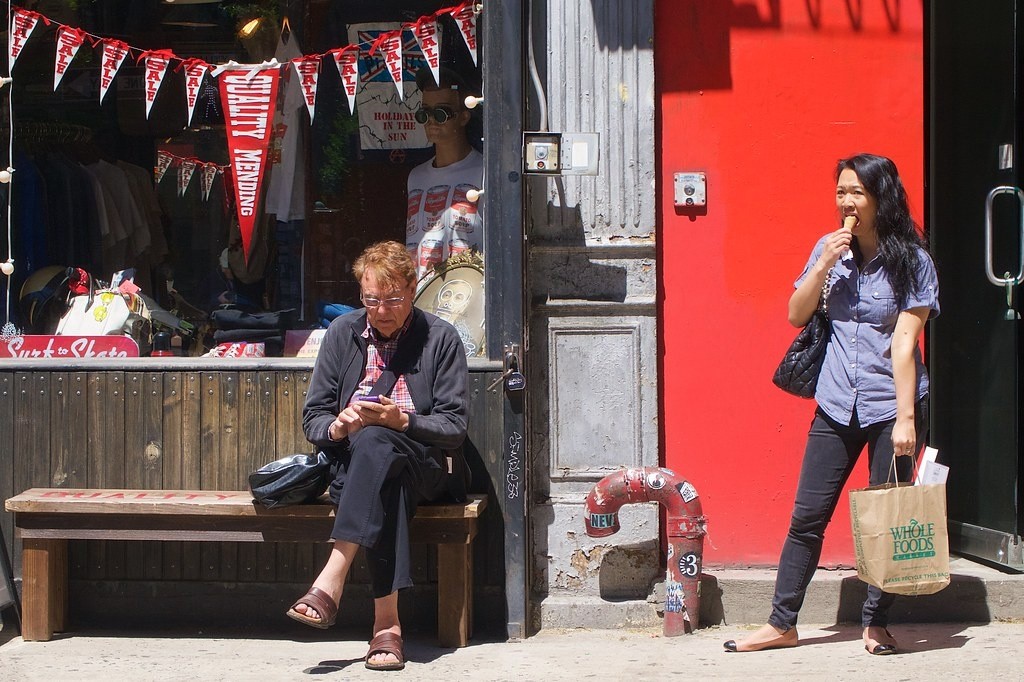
364 632 405 671
287 587 339 629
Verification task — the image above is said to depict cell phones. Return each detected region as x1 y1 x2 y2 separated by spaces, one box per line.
359 396 380 404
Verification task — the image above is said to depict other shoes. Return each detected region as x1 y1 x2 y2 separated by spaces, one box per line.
724 625 800 652
863 628 897 656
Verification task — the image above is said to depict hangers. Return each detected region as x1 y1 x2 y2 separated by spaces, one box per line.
13 120 116 163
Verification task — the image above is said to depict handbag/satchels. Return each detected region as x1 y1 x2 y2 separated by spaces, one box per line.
849 450 952 596
17 263 153 358
771 264 837 399
246 442 342 511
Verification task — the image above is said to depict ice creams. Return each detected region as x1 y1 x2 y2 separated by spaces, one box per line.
843 215 858 229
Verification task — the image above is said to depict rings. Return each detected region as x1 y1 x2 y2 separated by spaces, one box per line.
905 448 911 452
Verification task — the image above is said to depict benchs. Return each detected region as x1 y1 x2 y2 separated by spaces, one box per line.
6 488 490 649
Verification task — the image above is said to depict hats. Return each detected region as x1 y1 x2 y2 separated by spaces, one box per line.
415 47 478 100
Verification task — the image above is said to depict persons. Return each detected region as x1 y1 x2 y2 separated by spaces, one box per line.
286 59 486 671
722 153 941 655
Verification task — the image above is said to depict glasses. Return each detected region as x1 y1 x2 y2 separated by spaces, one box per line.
412 106 459 125
359 284 414 309
